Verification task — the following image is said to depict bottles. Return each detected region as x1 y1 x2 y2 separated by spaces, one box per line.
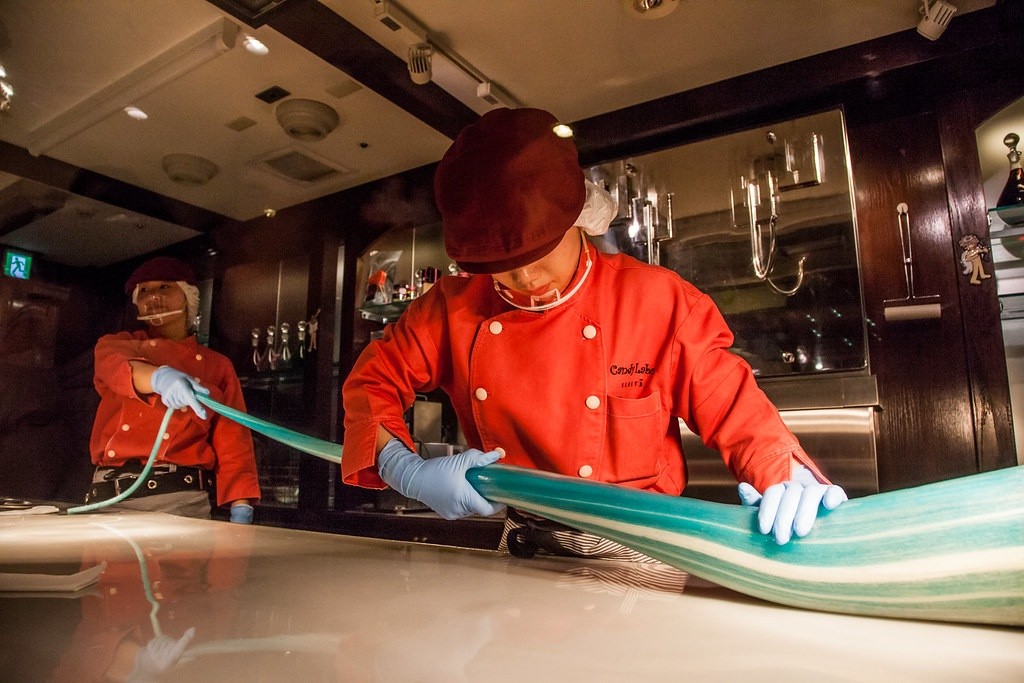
243 321 307 375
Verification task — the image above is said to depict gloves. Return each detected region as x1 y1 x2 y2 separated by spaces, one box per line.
230 504 253 524
150 364 210 420
377 437 507 521
738 464 849 544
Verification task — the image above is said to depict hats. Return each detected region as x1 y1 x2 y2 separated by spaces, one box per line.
434 108 586 275
125 256 194 297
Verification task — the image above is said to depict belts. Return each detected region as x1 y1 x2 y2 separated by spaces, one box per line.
84 468 213 503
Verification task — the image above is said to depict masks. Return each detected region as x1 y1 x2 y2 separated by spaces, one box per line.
136 294 185 325
494 230 592 311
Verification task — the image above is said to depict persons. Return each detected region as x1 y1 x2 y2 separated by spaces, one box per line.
88 246 257 528
336 107 848 592
